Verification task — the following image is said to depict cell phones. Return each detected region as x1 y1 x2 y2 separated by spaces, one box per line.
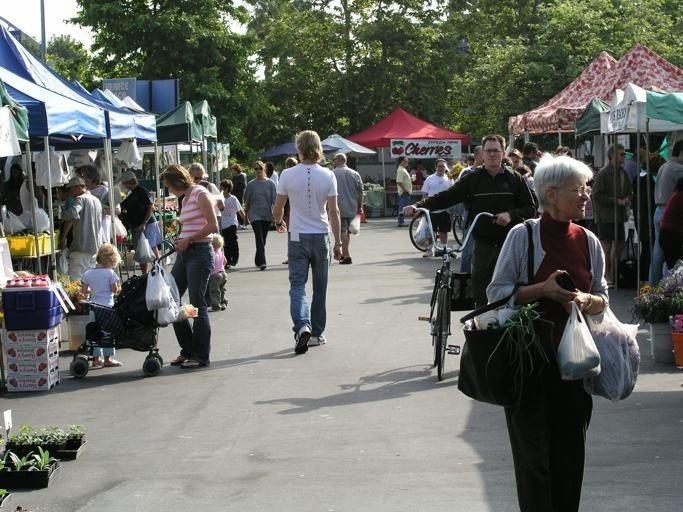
556 272 577 291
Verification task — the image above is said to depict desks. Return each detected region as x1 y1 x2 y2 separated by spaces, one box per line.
8 250 60 274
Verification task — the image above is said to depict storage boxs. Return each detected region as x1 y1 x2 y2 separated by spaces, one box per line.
3 329 61 393
6 229 60 257
2 284 74 330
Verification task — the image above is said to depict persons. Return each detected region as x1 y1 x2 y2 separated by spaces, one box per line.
273 129 343 356
1 161 247 370
241 155 364 270
395 133 682 315
484 153 610 512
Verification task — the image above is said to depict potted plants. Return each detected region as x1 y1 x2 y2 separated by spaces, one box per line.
0 424 89 493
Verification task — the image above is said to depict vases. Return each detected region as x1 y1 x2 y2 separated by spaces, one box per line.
672 333 682 372
650 323 673 365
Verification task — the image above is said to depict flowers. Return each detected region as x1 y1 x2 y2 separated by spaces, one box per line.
629 259 682 321
667 312 682 331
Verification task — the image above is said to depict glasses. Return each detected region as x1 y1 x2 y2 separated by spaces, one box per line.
550 185 592 196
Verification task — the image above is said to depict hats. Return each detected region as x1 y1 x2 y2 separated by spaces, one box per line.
122 170 135 183
65 177 87 189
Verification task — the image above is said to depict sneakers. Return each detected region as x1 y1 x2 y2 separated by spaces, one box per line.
180 360 202 368
207 309 216 312
170 356 188 365
423 251 433 257
221 304 226 310
309 335 325 346
339 257 352 264
92 360 104 367
294 326 311 354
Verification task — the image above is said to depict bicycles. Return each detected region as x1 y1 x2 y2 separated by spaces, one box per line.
409 209 465 252
399 207 508 383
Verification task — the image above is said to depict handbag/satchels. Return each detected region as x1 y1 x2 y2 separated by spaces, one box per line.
617 259 636 288
455 281 555 408
450 272 476 311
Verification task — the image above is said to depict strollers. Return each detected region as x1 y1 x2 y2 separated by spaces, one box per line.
67 239 175 378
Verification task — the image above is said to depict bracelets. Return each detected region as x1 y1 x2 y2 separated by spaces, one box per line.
584 293 592 309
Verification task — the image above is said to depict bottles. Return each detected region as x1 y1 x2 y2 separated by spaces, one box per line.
667 315 675 333
5 274 50 287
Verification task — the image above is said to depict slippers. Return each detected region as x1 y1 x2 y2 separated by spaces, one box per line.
104 359 122 366
